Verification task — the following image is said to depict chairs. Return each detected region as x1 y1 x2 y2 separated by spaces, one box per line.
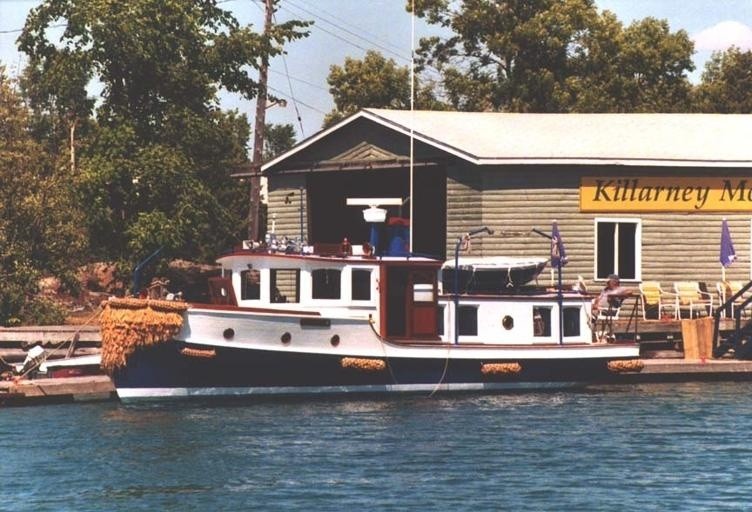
593 305 620 340
718 280 751 319
673 282 716 319
638 280 679 324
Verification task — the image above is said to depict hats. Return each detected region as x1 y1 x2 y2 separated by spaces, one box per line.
606 274 620 283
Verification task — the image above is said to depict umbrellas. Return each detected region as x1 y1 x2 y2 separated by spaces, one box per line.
720 221 738 317
549 219 567 285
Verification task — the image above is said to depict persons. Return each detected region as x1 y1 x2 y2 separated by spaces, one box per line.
594 275 632 313
19 340 47 378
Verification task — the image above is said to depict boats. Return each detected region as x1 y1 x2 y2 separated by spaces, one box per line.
95 192 651 410
0 350 105 377
